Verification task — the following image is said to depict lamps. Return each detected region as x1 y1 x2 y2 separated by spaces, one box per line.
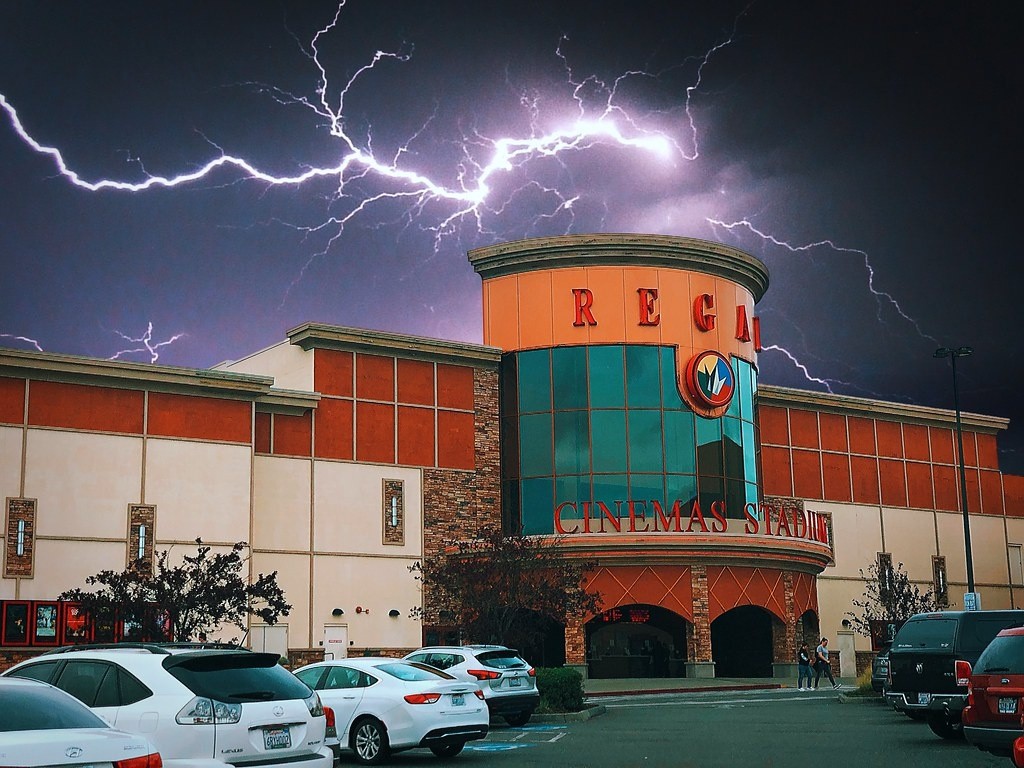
261 608 273 614
138 524 145 560
439 610 450 618
842 619 851 626
332 609 343 616
389 610 400 616
391 495 398 527
17 518 25 556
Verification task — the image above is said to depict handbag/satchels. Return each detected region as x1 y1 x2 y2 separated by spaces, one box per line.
812 657 825 670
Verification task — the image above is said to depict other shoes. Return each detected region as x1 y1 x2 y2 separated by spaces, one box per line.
833 684 842 690
807 687 815 691
798 688 806 691
814 687 822 691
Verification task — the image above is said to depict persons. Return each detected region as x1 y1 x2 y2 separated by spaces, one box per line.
797 637 842 692
195 632 214 649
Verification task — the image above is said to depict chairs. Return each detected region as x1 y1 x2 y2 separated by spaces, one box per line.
334 669 353 689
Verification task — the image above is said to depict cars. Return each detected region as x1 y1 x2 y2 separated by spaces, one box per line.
0 676 161 768
4 641 334 768
405 643 541 726
291 658 492 764
956 627 1024 768
871 608 1024 738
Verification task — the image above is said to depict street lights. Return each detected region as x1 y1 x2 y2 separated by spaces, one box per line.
933 343 980 608
